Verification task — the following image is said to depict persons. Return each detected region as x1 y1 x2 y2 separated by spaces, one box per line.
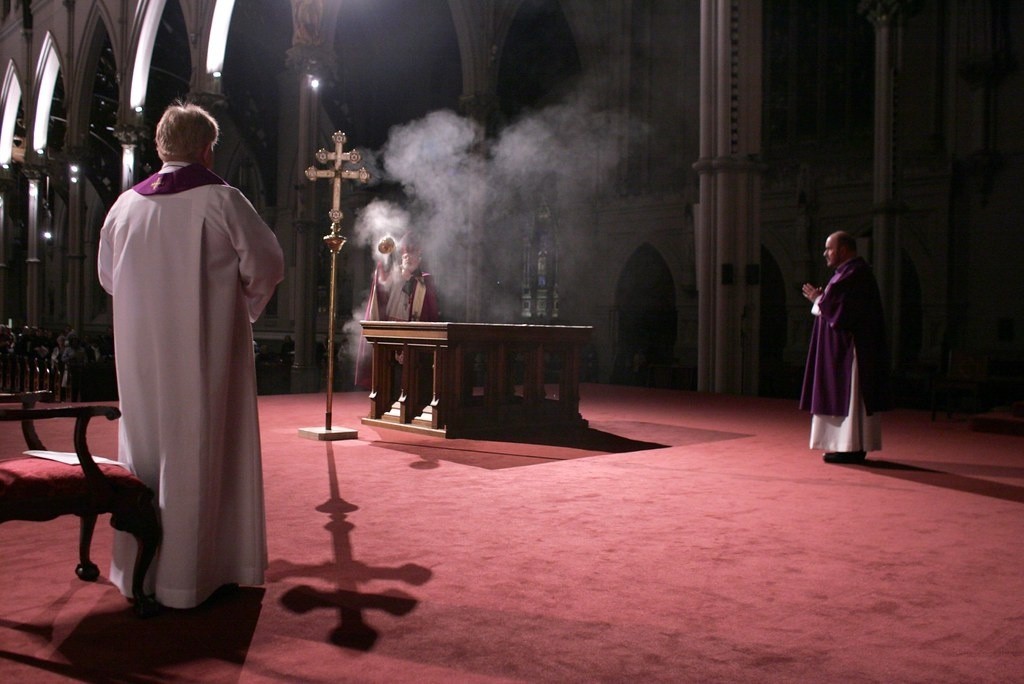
97 98 286 611
356 230 439 389
799 231 889 464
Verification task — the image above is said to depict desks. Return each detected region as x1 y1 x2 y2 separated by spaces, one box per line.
360 319 592 439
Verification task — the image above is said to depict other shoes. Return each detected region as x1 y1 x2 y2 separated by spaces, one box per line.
150 583 241 616
823 451 866 462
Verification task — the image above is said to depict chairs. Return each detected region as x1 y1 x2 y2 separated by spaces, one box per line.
0 390 164 623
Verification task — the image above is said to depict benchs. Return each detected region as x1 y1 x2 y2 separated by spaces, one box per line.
0 335 328 402
